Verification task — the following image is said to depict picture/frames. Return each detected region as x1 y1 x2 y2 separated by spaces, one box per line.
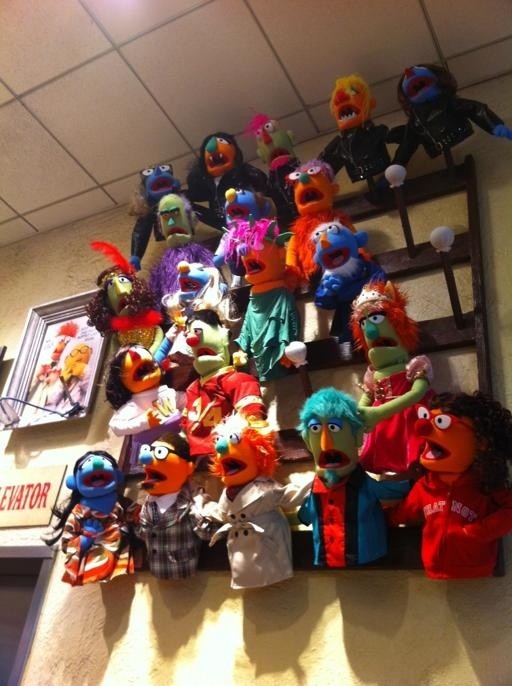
0 288 110 430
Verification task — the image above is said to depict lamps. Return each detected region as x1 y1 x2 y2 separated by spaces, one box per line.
0 397 84 427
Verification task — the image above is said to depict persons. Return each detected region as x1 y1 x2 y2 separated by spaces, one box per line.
40 54 512 594
